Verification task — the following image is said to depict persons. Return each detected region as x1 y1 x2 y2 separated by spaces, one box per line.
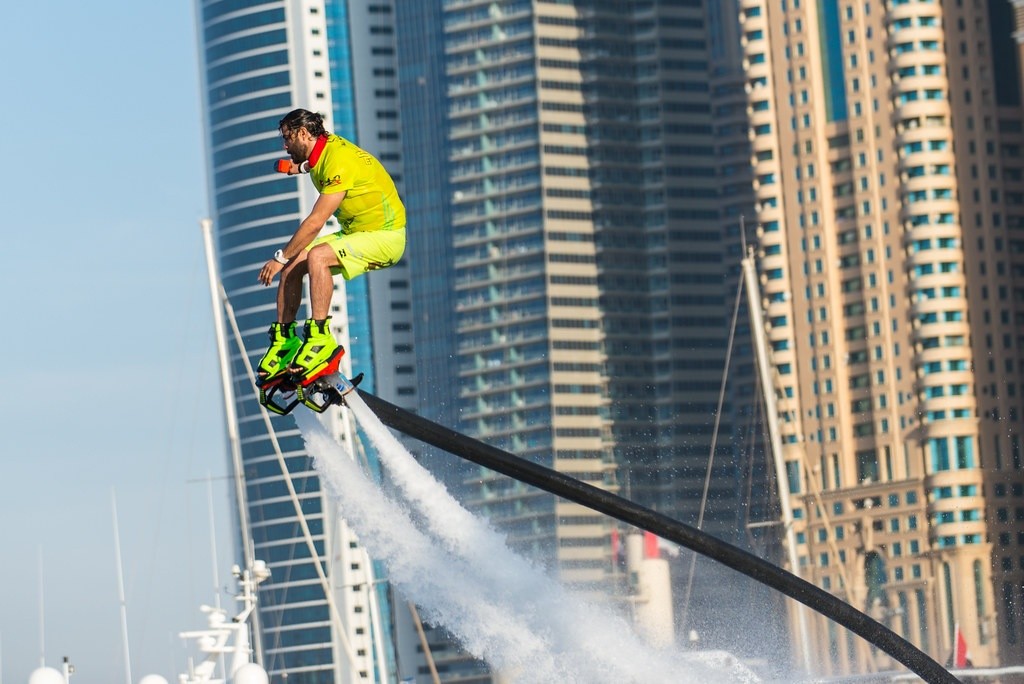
255 107 406 386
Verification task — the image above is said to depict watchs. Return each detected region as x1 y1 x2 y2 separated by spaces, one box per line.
274 249 289 264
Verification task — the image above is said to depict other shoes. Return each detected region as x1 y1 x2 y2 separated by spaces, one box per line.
285 316 338 378
252 322 303 381
